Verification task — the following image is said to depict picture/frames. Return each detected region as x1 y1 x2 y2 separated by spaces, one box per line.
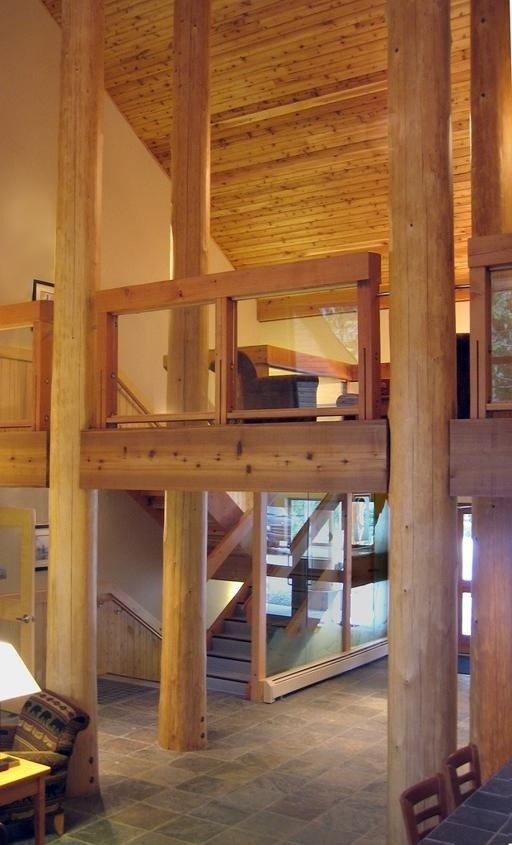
33 279 54 303
34 524 49 571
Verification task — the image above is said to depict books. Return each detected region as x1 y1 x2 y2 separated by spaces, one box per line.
0 753 20 772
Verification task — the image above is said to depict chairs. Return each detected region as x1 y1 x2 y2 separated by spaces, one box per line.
233 350 319 422
397 743 481 845
1 689 92 836
